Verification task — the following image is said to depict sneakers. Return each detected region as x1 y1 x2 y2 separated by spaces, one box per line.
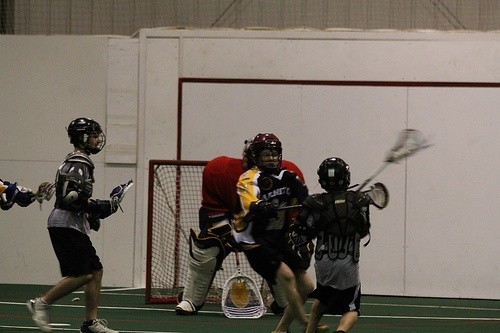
27 299 53 333
79 319 119 333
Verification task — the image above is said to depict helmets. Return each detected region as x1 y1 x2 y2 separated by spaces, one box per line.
250 133 283 174
67 117 103 155
317 157 350 192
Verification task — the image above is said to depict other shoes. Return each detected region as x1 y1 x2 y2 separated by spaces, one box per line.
316 325 330 333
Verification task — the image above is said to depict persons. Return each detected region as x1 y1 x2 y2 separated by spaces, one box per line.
28 118 128 333
176 133 370 333
0 177 45 210
